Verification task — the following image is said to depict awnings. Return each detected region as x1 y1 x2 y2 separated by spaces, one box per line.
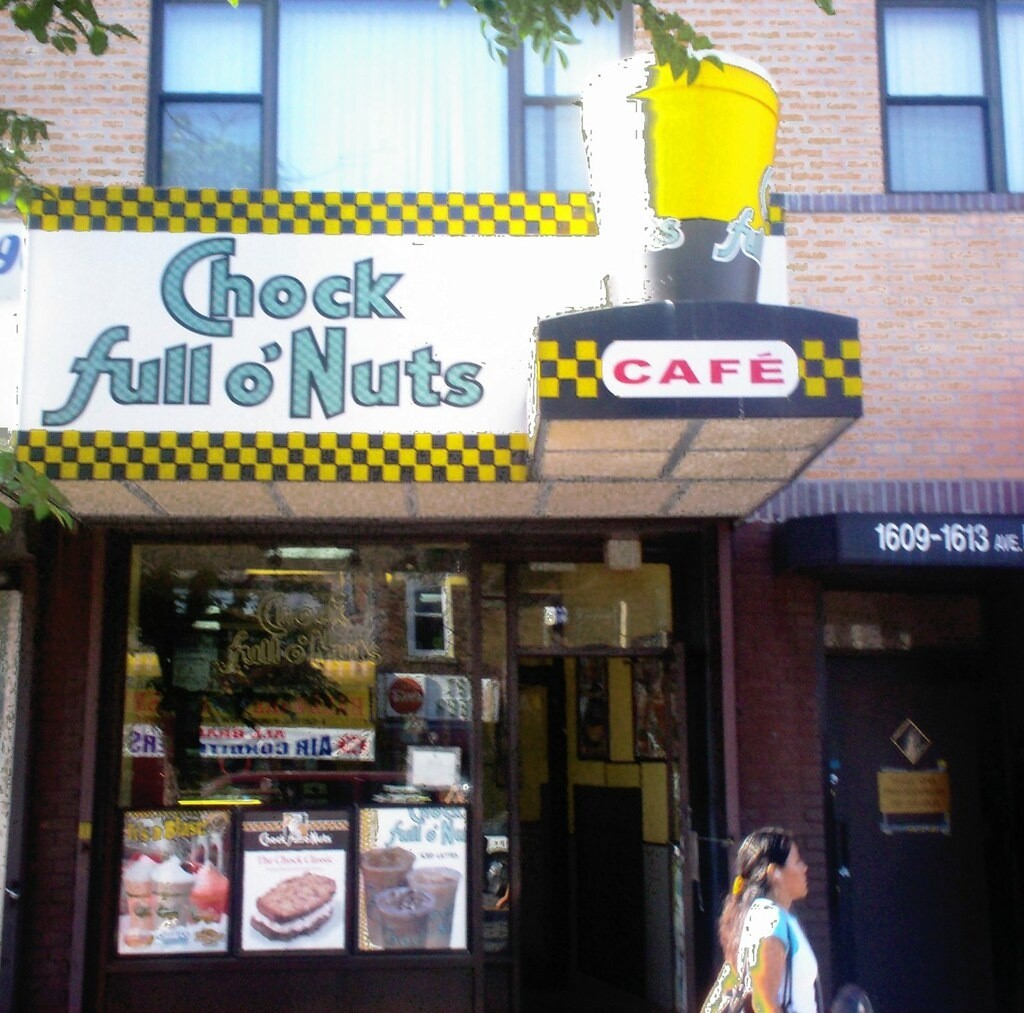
776 513 1024 573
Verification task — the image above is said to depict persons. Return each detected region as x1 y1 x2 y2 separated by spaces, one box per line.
721 828 818 1013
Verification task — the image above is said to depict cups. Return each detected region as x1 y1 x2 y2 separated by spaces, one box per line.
152 876 193 945
121 874 156 946
189 873 232 945
361 847 416 945
375 888 434 950
408 867 461 949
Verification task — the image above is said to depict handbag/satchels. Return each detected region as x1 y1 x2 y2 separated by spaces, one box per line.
699 960 744 1013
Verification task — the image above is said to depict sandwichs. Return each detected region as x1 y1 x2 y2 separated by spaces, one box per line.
251 873 338 941
